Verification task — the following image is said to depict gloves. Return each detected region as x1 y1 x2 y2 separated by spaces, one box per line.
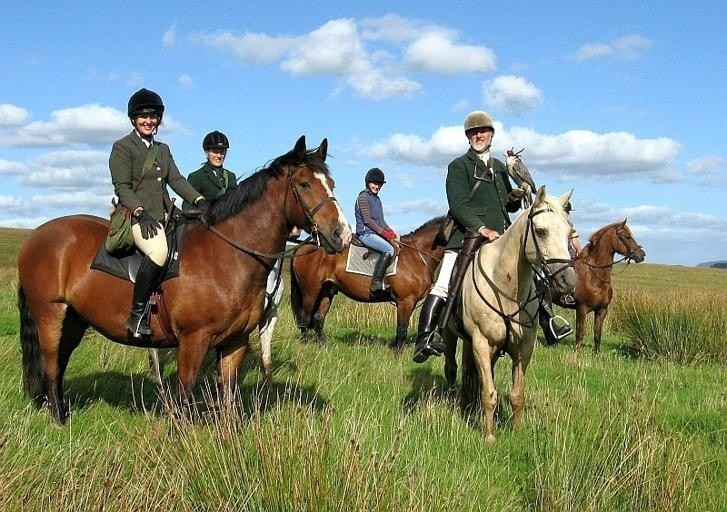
135 209 162 239
380 228 397 239
197 199 207 207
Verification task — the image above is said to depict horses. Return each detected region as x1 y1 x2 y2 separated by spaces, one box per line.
549 217 646 357
434 184 578 446
16 135 354 440
290 215 448 365
148 224 301 397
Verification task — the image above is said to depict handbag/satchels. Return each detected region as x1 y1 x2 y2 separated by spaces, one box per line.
431 210 458 250
105 205 134 257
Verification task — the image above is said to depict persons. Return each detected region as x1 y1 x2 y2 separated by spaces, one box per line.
354 168 397 303
553 199 582 309
414 111 571 364
108 88 206 336
182 131 237 211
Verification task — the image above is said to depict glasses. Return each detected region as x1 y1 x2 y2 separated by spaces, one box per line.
473 165 495 183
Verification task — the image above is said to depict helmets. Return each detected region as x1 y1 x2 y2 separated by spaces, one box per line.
365 168 386 183
464 111 495 132
203 131 229 149
128 88 164 116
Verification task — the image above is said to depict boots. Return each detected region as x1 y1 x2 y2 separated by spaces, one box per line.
369 251 395 293
413 294 448 364
125 254 164 336
539 286 574 344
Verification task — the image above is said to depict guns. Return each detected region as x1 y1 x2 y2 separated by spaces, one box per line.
440 230 480 332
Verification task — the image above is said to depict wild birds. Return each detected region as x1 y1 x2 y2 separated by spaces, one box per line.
503 147 538 210
